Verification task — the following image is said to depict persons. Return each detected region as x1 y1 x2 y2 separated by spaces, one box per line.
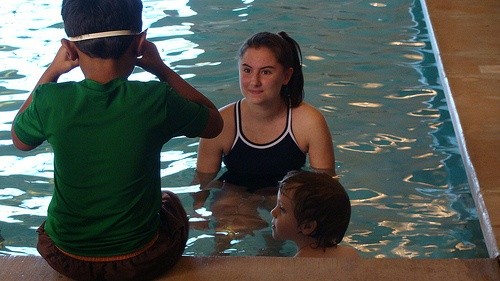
189 31 336 257
13 0 223 280
272 168 357 258
